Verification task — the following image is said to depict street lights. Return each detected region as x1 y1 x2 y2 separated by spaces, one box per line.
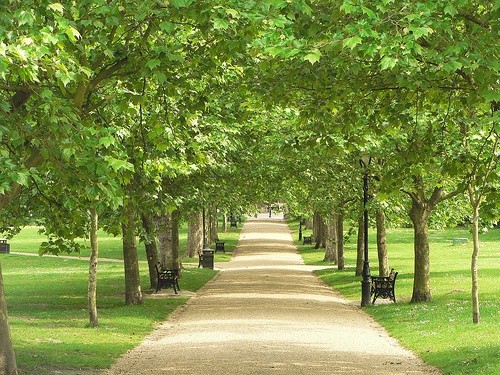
359 153 373 307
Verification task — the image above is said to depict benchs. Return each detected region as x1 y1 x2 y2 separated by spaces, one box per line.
154 262 180 295
371 269 397 304
198 251 203 268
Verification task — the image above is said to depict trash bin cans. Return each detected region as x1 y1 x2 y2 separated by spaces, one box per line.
202 249 214 271
0 239 10 255
